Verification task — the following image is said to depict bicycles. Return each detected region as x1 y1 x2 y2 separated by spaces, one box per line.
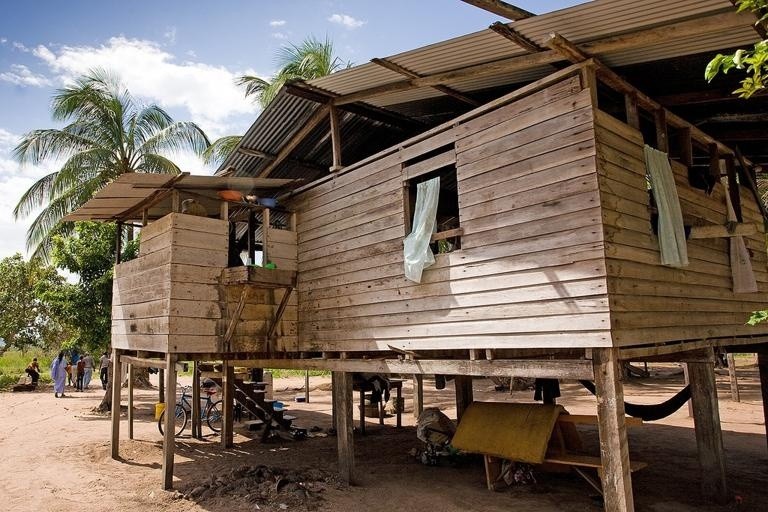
156 382 240 438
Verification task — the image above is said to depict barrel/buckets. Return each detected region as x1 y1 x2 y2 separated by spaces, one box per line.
392 396 404 413
154 402 166 421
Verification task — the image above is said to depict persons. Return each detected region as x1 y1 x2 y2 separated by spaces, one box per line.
23 349 109 392
49 351 68 398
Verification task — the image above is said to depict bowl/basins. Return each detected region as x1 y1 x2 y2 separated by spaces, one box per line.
217 190 245 203
256 197 279 208
